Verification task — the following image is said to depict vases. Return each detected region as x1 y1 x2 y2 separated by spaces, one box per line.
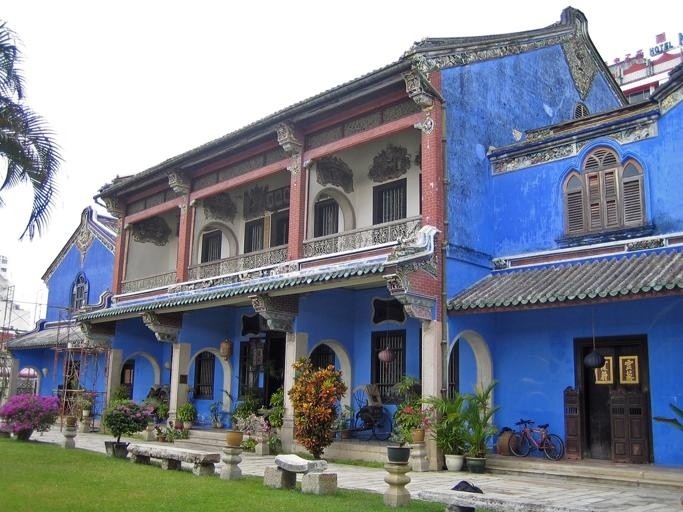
67 416 76 424
83 410 90 417
16 429 33 440
105 441 130 458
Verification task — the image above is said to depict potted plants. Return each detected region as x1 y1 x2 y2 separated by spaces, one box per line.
387 377 502 473
209 401 223 427
226 415 258 452
155 399 195 442
329 405 354 439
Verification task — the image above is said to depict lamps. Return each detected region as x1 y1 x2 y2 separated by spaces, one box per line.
220 337 231 361
584 301 606 368
378 295 396 362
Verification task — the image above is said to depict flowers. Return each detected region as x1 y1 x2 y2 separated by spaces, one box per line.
78 392 96 410
0 394 60 436
104 401 154 442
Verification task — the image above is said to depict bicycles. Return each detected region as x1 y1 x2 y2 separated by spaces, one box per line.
508 419 564 462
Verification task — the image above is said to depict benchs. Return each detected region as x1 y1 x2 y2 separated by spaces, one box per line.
263 454 337 495
127 443 220 476
418 490 598 512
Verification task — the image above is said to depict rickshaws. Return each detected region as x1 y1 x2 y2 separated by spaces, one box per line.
338 385 392 442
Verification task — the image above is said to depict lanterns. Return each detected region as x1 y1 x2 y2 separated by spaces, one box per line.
583 351 605 368
220 339 233 361
378 347 396 364
